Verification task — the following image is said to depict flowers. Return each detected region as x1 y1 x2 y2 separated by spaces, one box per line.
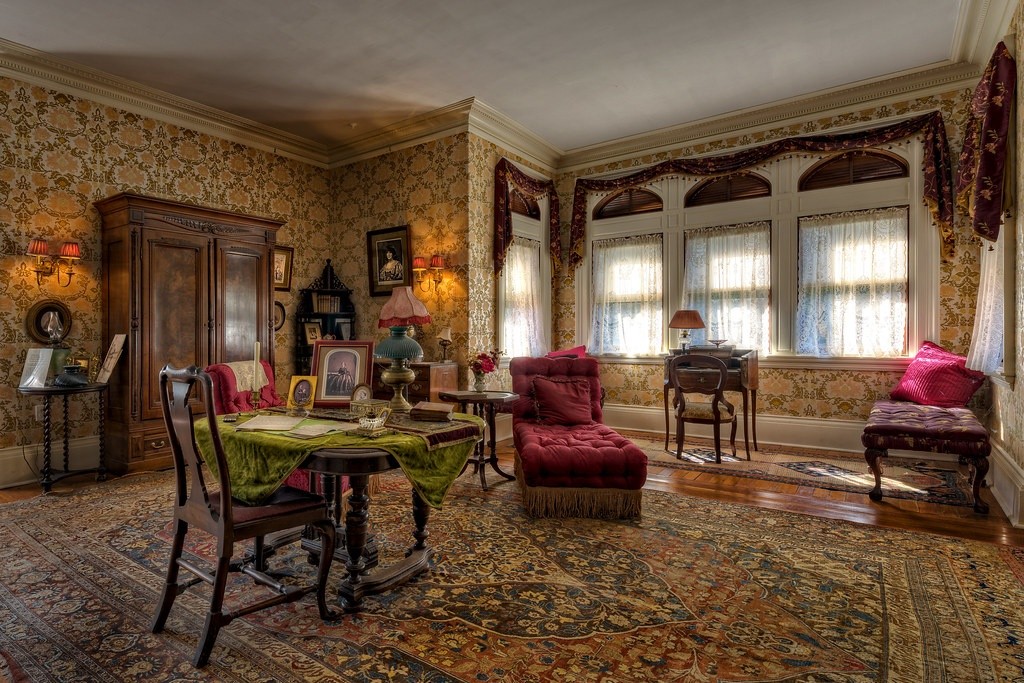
467 345 504 376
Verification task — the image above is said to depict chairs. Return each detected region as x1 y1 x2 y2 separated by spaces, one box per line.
668 353 738 463
140 361 338 668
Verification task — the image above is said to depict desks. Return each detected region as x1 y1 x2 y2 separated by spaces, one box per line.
18 380 112 495
662 349 759 460
189 402 485 613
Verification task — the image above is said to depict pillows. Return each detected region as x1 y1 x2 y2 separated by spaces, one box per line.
532 372 592 426
892 337 989 403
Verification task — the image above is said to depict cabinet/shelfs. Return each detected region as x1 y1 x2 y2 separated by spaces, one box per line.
91 191 287 477
293 257 356 376
373 361 459 409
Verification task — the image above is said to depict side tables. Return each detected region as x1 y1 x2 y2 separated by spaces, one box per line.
437 388 520 494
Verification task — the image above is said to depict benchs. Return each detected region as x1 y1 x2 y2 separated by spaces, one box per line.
861 402 994 515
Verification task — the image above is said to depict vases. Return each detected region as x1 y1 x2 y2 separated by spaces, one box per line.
473 374 487 392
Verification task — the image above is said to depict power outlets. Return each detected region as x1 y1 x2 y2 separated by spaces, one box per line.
34 405 46 421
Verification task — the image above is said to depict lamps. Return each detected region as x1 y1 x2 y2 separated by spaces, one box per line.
26 237 82 288
374 252 454 416
668 310 707 355
45 311 72 377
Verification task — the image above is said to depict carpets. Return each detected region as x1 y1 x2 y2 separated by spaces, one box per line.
618 429 975 504
0 454 1024 683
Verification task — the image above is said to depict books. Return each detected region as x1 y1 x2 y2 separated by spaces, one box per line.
409 400 454 422
313 293 341 313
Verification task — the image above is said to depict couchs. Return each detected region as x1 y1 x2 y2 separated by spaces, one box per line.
205 356 385 507
509 354 648 521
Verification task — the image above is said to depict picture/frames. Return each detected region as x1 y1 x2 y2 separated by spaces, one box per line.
273 300 286 331
26 297 73 343
286 374 317 410
72 356 91 378
303 321 323 346
309 339 372 409
366 223 414 297
274 244 295 291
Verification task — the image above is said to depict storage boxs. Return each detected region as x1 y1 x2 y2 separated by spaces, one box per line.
350 398 390 415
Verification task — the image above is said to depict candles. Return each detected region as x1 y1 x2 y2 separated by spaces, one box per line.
253 340 260 391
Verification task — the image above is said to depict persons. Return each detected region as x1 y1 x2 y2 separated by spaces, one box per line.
297 384 307 404
328 361 353 396
379 244 402 281
